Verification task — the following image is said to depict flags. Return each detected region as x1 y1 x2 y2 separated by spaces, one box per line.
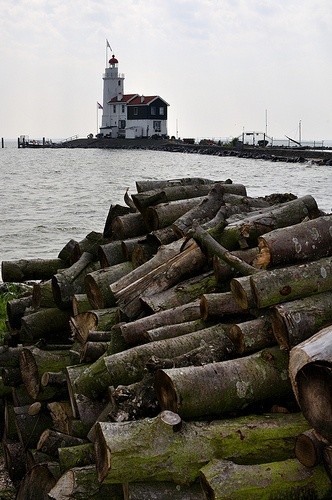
97 102 103 109
106 40 112 51
